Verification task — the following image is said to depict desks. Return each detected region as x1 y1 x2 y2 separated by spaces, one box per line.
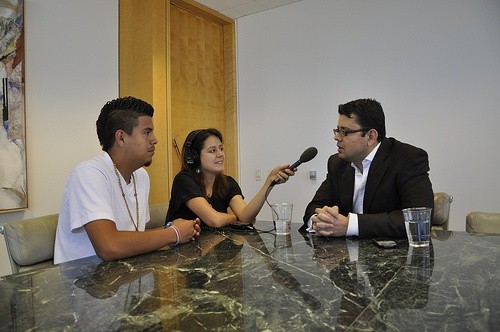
0 221 500 332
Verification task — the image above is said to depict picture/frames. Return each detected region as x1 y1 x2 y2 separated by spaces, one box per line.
0 0 30 213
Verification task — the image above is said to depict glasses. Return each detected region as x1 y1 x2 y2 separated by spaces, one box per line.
332 128 370 137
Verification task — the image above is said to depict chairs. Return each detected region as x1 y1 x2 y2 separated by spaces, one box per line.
146 205 169 229
431 193 454 232
0 214 59 274
466 212 500 234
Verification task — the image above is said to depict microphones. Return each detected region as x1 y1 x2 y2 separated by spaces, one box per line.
269 147 318 186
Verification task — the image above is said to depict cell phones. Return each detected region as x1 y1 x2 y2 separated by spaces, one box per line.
373 238 397 249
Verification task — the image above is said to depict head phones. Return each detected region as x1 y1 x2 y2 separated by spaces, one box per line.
184 129 203 174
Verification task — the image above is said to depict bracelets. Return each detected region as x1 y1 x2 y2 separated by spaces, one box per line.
171 225 181 246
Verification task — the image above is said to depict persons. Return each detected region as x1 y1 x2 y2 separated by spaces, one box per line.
54 96 200 262
309 238 435 332
62 244 202 332
175 226 300 291
303 99 435 237
165 128 298 227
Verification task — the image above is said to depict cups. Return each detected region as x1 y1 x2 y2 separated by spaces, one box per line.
271 203 293 234
405 246 431 288
402 207 433 248
272 235 296 264
